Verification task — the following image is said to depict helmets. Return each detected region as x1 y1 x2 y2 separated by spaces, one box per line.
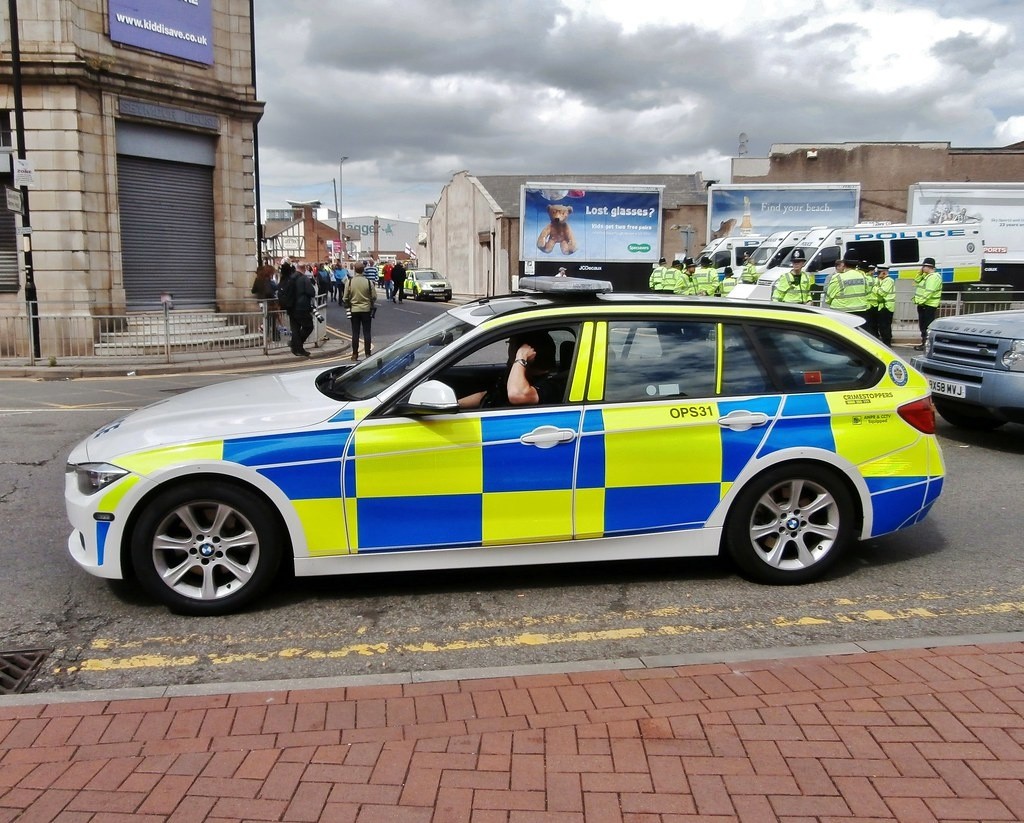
684 256 697 268
670 260 681 267
790 249 806 261
723 268 734 276
842 248 859 263
920 258 935 266
659 257 666 264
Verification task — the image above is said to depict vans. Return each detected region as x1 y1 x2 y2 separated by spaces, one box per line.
683 219 986 314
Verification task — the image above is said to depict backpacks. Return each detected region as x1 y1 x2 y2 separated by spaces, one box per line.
277 273 303 310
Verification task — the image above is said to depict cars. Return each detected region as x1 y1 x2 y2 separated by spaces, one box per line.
400 268 453 302
59 273 949 618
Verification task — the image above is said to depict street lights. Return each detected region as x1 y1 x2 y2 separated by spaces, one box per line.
338 154 349 267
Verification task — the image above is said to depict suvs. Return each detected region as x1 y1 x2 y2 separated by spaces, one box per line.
908 308 1024 435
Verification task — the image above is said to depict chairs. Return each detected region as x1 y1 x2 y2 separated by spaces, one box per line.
560 342 576 378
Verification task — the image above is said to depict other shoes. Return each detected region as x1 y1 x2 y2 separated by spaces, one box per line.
291 347 302 356
386 296 404 304
300 349 310 356
258 324 264 333
333 297 337 302
338 302 342 306
330 297 333 302
915 344 924 350
351 357 357 361
366 353 371 358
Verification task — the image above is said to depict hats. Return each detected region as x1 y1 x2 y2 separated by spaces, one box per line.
698 256 712 265
866 264 875 270
876 264 889 271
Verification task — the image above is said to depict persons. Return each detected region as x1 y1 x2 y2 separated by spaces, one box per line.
771 248 812 306
313 259 354 307
648 254 719 297
822 247 895 349
457 332 561 411
250 255 316 358
377 261 407 305
718 266 740 298
742 250 758 283
363 260 378 287
341 260 378 361
911 258 942 350
555 267 567 277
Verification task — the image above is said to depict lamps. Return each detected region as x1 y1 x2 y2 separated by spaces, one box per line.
807 150 818 159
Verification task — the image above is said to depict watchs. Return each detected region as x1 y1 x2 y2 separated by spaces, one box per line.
514 359 528 366
